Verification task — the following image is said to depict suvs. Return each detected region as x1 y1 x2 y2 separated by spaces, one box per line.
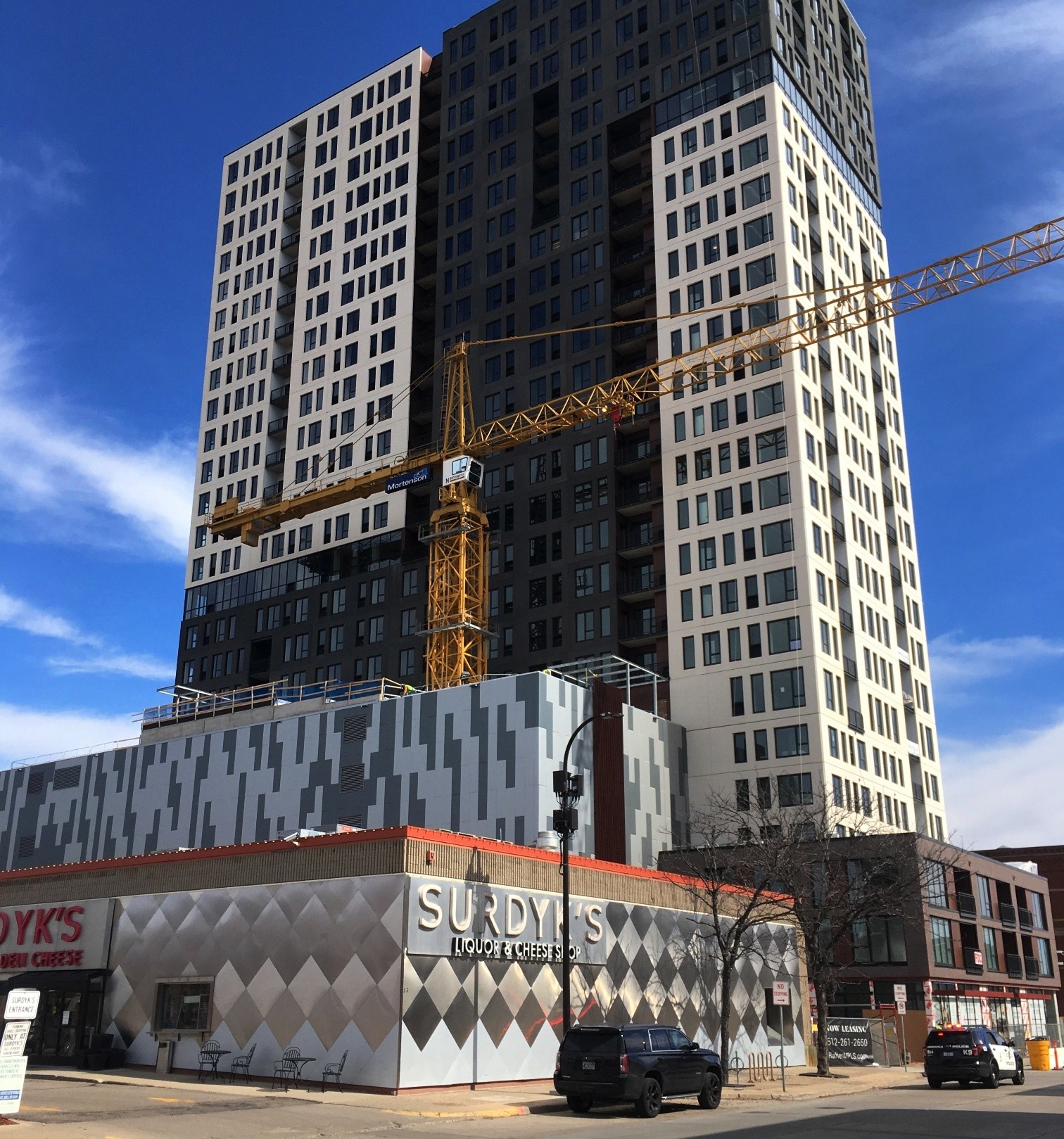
922 1024 1026 1089
553 1022 723 1118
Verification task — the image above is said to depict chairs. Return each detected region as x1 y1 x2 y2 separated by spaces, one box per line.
198 1040 221 1079
229 1043 257 1084
321 1050 350 1094
271 1046 301 1090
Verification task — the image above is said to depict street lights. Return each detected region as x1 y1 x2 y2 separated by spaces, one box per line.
557 712 625 1040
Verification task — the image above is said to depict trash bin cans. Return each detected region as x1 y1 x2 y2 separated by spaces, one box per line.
156 1041 173 1073
1026 1037 1050 1070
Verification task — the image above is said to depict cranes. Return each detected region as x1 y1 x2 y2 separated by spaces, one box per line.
210 214 1063 691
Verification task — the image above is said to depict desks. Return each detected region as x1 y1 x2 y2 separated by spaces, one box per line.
284 1058 316 1093
199 1051 231 1084
